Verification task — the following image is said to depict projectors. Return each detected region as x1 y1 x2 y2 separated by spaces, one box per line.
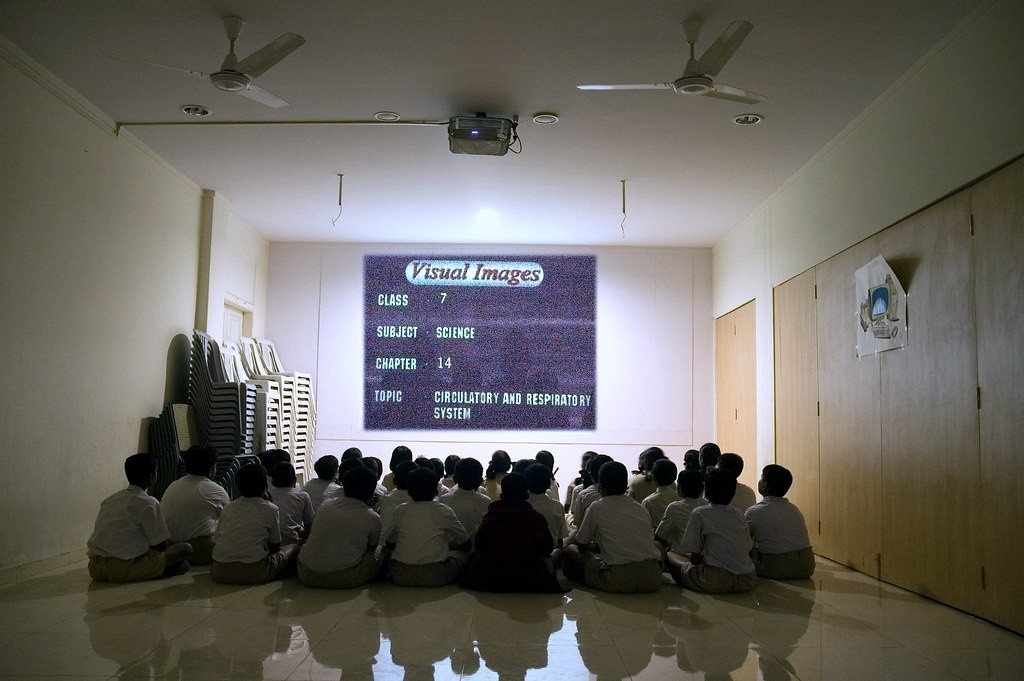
446 116 512 155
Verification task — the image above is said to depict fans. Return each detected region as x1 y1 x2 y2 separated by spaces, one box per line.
102 17 305 110
576 19 767 107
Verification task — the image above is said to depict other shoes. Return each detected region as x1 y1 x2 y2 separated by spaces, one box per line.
166 560 189 575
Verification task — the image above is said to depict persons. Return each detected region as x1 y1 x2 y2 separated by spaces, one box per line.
84 442 815 594
82 577 816 681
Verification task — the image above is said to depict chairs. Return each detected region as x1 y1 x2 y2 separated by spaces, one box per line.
151 330 318 502
